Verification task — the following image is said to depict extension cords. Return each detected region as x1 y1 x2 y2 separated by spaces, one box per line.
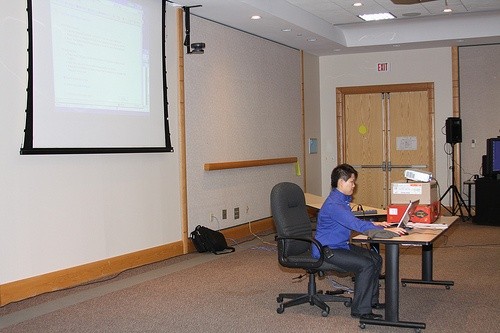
353 209 377 215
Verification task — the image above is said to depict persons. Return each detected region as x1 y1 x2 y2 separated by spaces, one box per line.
312 164 407 320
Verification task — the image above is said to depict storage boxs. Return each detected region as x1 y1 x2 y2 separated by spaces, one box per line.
391 178 437 204
387 201 440 223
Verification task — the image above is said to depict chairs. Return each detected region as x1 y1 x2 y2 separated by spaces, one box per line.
271 182 352 317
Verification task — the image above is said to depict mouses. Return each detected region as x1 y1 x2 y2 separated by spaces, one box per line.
401 232 409 235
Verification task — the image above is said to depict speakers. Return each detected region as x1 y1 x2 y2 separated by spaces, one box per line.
446 117 462 143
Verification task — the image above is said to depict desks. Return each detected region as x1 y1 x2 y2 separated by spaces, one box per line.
351 216 460 333
464 180 476 221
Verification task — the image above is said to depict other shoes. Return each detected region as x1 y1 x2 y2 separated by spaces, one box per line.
372 303 383 309
351 312 382 320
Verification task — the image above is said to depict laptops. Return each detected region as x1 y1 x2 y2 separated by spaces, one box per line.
383 199 419 228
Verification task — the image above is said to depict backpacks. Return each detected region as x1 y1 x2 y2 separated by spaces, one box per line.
191 225 235 256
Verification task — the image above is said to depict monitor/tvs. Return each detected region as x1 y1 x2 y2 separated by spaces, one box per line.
487 138 500 173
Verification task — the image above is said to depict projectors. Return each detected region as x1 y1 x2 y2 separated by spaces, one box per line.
404 168 433 182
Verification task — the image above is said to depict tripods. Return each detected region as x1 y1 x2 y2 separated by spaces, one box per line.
439 144 473 222
